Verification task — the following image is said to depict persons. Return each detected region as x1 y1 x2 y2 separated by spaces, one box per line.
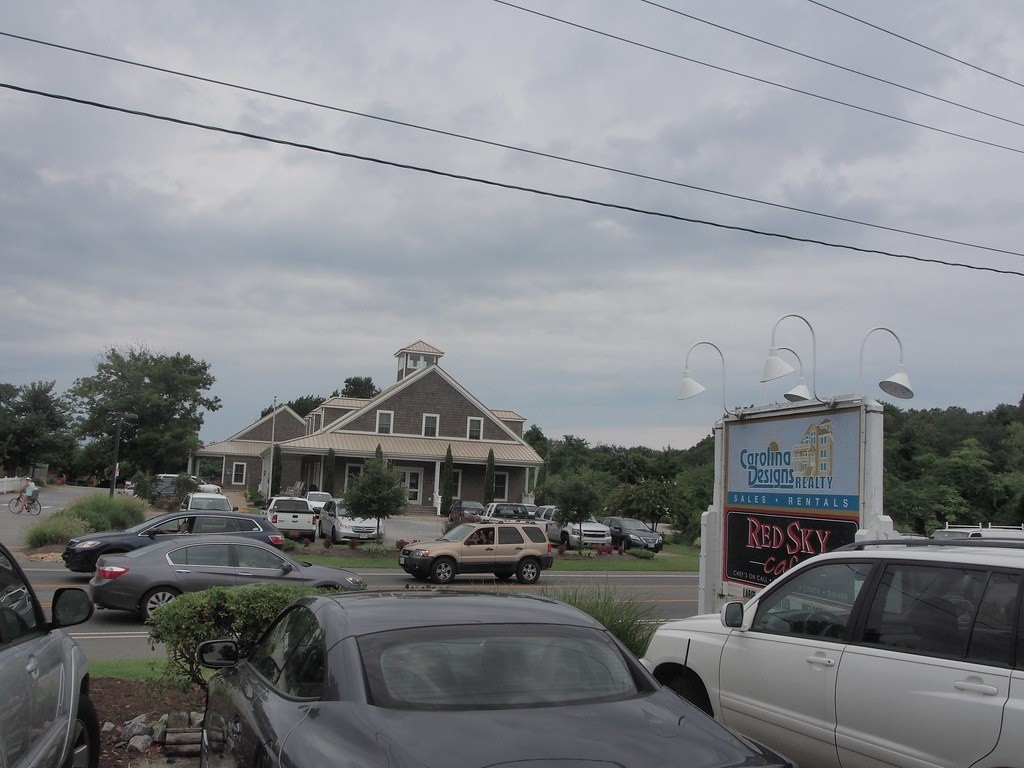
18 477 35 513
158 522 189 534
469 531 491 544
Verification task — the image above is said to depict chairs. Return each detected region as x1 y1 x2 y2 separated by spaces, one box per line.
911 596 961 656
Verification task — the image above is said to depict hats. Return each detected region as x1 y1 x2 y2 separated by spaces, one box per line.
25 477 32 481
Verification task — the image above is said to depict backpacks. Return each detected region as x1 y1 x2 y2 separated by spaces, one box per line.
31 488 40 499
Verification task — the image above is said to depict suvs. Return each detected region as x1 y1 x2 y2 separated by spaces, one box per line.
534 505 613 550
61 510 290 574
637 535 1024 768
398 522 554 585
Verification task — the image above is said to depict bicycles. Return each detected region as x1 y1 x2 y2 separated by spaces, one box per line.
8 487 43 517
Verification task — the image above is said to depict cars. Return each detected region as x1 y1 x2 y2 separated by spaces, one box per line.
1 543 101 768
302 491 333 517
147 472 233 511
601 516 663 553
446 498 550 535
320 498 385 544
196 587 801 768
90 533 369 624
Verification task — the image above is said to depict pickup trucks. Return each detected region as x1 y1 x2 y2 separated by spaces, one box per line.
261 497 318 542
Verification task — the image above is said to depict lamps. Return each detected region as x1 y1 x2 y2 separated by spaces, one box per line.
761 313 836 407
853 327 916 401
777 346 811 401
677 341 739 416
